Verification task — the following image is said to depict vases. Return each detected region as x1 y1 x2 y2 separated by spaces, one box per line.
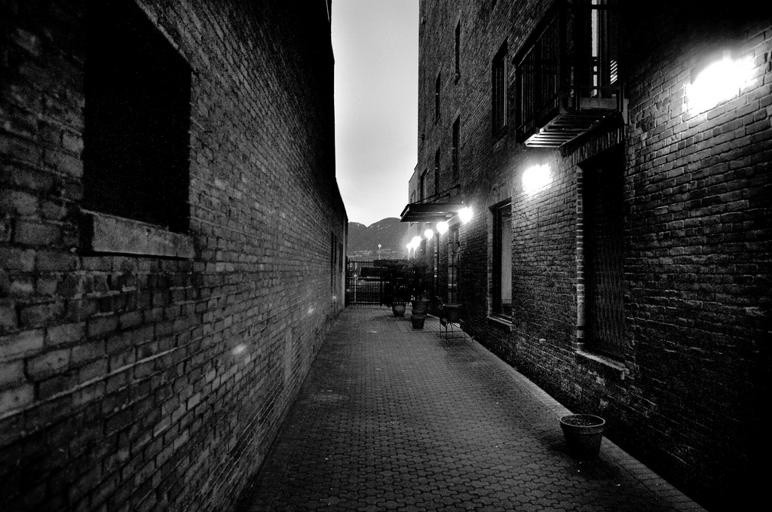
561 414 606 458
